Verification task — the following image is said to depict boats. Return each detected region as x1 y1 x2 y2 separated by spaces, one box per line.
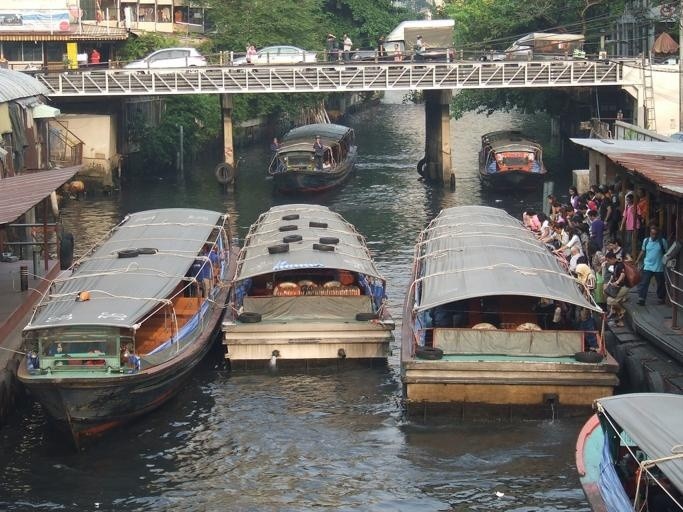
264 123 357 197
221 204 393 366
573 390 683 512
18 208 228 445
399 204 620 408
478 130 548 191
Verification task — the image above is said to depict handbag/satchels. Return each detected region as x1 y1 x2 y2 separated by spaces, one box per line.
604 284 620 298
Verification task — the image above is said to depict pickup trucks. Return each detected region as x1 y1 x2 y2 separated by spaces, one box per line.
351 40 455 67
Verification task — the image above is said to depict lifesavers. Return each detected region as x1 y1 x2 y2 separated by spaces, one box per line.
323 280 344 288
472 322 498 331
297 280 319 288
273 282 300 296
516 322 542 330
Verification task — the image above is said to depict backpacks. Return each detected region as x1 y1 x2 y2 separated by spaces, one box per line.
623 261 640 287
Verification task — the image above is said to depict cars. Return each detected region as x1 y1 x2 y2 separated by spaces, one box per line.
229 45 317 73
118 47 208 77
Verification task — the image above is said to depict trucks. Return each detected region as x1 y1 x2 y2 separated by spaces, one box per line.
377 20 456 63
502 31 587 68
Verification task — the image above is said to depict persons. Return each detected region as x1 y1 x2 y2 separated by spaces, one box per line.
635 226 649 250
270 137 281 173
244 42 256 63
635 226 669 306
634 183 649 226
392 43 403 61
90 48 101 64
325 33 338 61
313 135 325 171
343 34 354 62
323 159 331 168
523 184 634 352
616 109 623 119
415 35 430 61
